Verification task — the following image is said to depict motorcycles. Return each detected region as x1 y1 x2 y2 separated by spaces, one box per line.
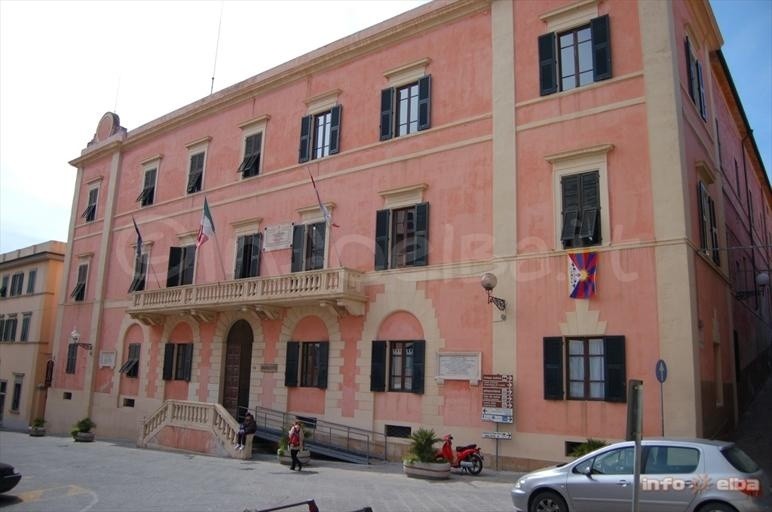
434 434 484 474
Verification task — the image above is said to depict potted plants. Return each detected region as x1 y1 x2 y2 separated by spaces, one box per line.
401 426 453 481
276 420 314 467
27 416 50 436
69 416 98 442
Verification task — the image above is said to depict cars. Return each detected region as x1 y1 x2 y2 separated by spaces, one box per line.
512 438 772 512
0 462 22 496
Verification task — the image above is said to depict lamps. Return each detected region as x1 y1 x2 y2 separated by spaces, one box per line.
71 325 93 351
730 271 767 301
480 271 506 312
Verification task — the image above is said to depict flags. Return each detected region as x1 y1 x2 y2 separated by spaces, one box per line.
311 176 340 227
195 194 216 247
566 247 599 299
132 219 148 256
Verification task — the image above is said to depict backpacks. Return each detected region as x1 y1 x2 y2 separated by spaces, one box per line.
291 426 300 447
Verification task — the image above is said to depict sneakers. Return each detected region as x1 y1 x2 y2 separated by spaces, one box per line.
239 445 245 450
235 444 241 449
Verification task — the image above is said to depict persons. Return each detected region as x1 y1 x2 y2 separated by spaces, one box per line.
288 421 305 472
233 412 257 450
